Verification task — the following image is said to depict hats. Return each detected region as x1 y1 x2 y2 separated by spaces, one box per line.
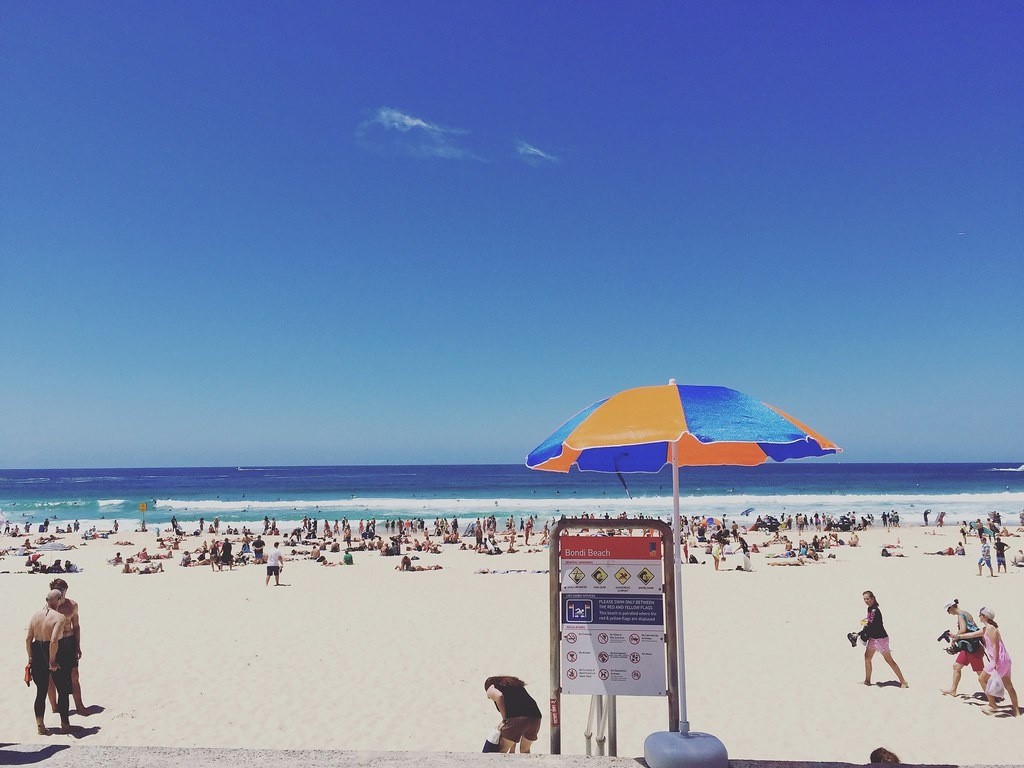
980 607 995 619
944 599 956 611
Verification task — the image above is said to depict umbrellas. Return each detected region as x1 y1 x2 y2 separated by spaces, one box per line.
527 380 841 732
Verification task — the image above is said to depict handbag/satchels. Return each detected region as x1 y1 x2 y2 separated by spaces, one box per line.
482 729 501 753
986 669 1004 697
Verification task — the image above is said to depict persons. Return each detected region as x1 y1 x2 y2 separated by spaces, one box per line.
0 510 1024 586
46 578 90 715
850 590 908 690
26 589 80 736
946 606 1021 717
484 675 541 752
939 599 990 697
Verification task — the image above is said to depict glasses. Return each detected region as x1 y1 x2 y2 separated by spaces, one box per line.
53 587 65 591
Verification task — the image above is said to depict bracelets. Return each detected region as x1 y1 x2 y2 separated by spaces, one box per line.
960 634 962 639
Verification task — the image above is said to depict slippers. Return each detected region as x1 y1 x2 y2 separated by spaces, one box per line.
847 632 857 647
938 630 951 643
